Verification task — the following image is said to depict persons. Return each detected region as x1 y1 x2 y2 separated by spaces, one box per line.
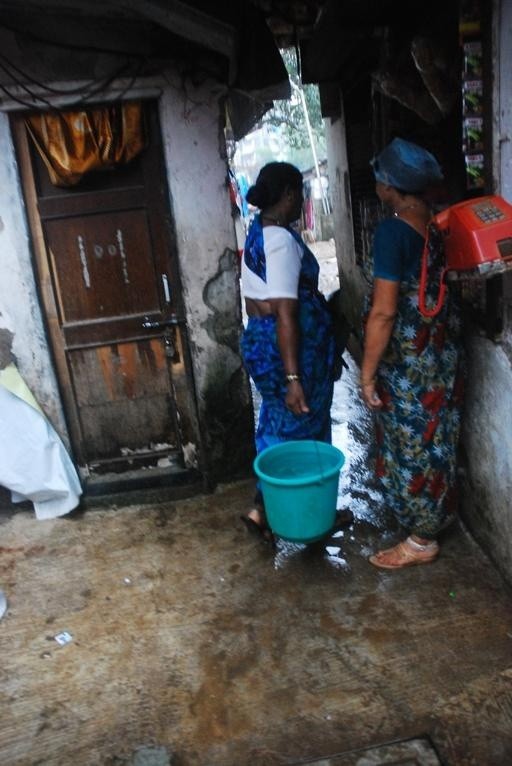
361 138 469 570
240 161 355 548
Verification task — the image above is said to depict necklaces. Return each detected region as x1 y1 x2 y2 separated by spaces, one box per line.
261 214 283 226
393 199 424 217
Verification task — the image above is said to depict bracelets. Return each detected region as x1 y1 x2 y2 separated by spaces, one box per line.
285 374 300 383
360 375 378 385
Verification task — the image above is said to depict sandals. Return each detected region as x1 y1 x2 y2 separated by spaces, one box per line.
369 537 438 570
241 504 276 544
305 509 353 547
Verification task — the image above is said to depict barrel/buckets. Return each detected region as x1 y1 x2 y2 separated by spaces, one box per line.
251 440 344 543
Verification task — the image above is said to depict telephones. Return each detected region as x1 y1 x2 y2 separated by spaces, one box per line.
432 195 510 269
